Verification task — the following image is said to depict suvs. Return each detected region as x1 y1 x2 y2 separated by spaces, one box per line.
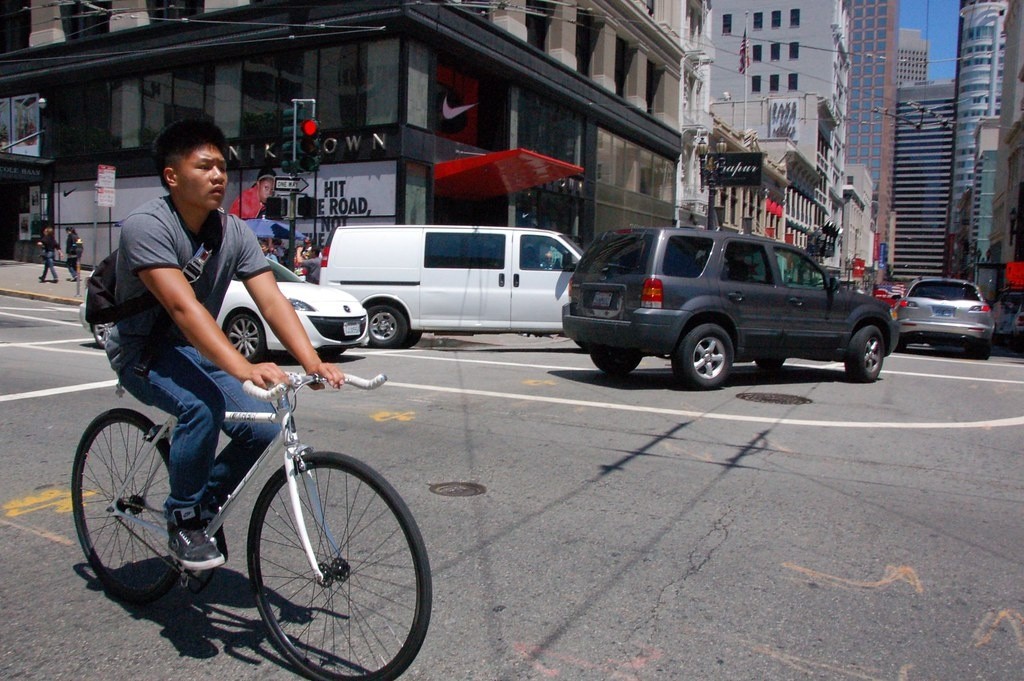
561 230 900 390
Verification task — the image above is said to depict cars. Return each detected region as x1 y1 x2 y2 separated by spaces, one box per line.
79 256 369 364
895 276 1024 360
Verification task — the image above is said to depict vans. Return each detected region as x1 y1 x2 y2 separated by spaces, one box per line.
320 225 584 349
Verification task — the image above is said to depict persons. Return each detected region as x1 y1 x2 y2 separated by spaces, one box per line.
105 118 345 572
258 238 322 286
986 249 994 262
977 248 981 262
65 226 83 281
37 228 64 283
229 167 278 217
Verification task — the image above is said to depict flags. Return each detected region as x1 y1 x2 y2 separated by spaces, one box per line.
738 27 750 74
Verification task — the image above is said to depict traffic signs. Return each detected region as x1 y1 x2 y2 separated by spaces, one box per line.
273 176 309 192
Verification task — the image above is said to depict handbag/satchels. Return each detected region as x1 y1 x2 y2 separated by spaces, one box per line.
85 204 222 324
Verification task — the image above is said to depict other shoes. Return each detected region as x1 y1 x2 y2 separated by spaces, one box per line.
38 276 45 282
70 275 78 281
53 280 58 283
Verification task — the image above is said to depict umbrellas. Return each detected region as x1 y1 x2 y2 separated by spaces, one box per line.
243 215 308 241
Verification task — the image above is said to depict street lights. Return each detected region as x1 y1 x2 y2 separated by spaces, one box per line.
698 138 728 229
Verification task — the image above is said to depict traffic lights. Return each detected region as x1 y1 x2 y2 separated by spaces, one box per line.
280 108 321 173
265 197 287 217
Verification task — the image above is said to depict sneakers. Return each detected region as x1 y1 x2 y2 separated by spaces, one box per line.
167 503 226 569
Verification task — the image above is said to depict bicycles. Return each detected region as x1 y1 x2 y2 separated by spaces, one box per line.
70 374 432 681
